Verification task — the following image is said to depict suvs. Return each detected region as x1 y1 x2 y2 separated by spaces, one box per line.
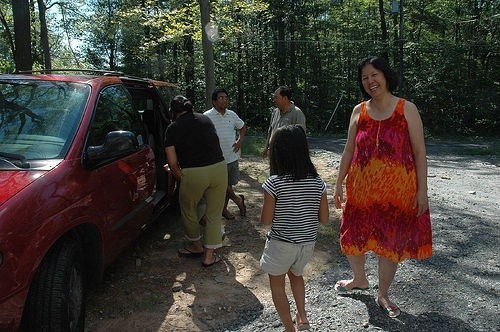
0 68 181 332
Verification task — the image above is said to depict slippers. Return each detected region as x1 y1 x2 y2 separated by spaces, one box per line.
375 297 402 319
222 211 235 220
202 254 223 267
178 247 205 258
238 195 247 217
334 279 371 292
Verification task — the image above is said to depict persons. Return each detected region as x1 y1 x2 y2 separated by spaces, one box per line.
333 56 432 319
203 87 246 220
164 95 228 266
260 125 328 332
262 86 305 176
